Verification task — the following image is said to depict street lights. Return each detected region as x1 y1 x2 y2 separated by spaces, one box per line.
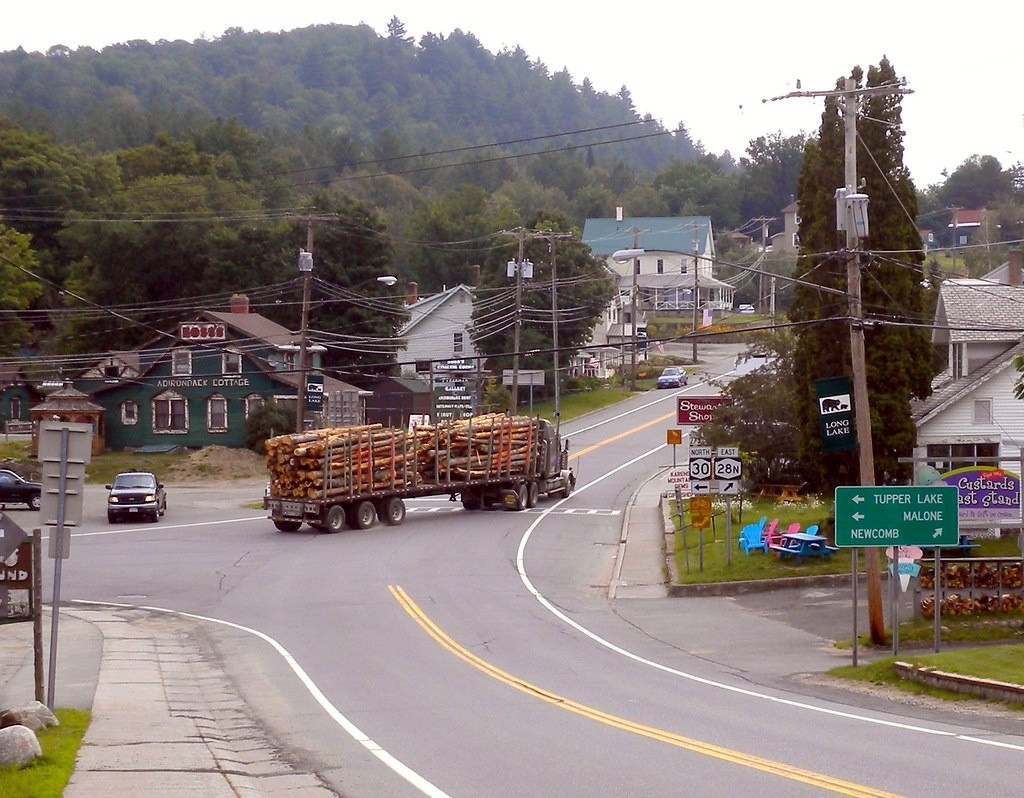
610 246 886 646
296 276 398 430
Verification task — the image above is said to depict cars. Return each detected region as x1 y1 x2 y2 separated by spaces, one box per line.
656 366 689 389
0 468 42 511
737 304 756 315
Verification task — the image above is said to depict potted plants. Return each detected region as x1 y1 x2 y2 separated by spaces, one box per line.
756 475 806 503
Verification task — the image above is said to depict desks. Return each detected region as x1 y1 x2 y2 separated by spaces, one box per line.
782 533 830 561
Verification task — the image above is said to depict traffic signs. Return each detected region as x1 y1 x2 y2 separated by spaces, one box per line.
836 486 961 546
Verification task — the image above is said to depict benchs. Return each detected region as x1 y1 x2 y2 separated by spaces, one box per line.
770 544 800 561
811 543 839 560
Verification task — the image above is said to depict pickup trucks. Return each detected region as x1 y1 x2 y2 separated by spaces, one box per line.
105 472 166 524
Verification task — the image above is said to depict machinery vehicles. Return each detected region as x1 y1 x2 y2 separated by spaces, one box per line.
263 411 577 536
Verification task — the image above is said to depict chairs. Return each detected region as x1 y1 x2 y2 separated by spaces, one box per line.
737 515 819 556
